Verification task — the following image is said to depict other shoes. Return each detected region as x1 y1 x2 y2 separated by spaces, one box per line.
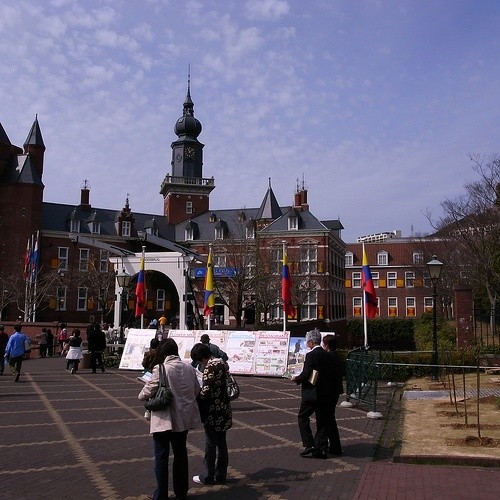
0 351 106 383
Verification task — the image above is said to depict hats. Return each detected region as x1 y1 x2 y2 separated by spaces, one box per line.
200 334 210 342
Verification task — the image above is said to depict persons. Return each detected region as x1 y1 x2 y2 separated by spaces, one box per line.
291 328 330 459
35 328 54 359
58 323 68 354
4 324 31 383
61 329 84 375
137 338 201 500
197 334 228 372
0 325 10 376
141 337 164 421
61 313 204 359
190 343 235 486
312 335 345 460
86 323 107 374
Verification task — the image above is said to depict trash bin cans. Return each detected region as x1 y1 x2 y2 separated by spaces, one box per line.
77 350 92 370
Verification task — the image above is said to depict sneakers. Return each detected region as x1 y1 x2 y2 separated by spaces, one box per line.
192 475 212 484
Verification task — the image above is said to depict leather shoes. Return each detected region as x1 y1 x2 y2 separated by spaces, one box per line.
300 445 327 458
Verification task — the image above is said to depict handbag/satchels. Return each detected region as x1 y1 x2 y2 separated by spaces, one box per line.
143 387 172 410
225 380 239 400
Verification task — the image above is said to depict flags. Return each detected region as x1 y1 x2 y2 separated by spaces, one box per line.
22 229 40 283
360 241 379 318
134 249 146 319
279 246 296 320
203 247 217 317
